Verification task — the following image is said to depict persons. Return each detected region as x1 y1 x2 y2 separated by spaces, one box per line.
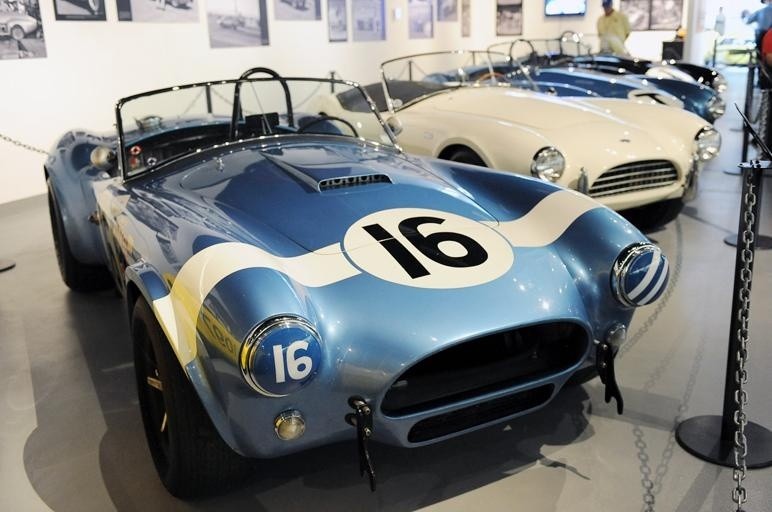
741 0 772 75
713 5 728 39
597 0 631 54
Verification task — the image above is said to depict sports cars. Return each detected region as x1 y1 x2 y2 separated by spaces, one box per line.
419 38 727 127
42 66 671 498
320 50 722 222
482 31 729 102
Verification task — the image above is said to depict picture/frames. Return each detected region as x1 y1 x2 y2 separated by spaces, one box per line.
495 0 523 37
648 1 685 32
619 1 649 31
53 0 108 20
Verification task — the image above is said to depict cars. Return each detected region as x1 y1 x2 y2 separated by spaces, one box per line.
0 1 38 40
700 31 759 66
